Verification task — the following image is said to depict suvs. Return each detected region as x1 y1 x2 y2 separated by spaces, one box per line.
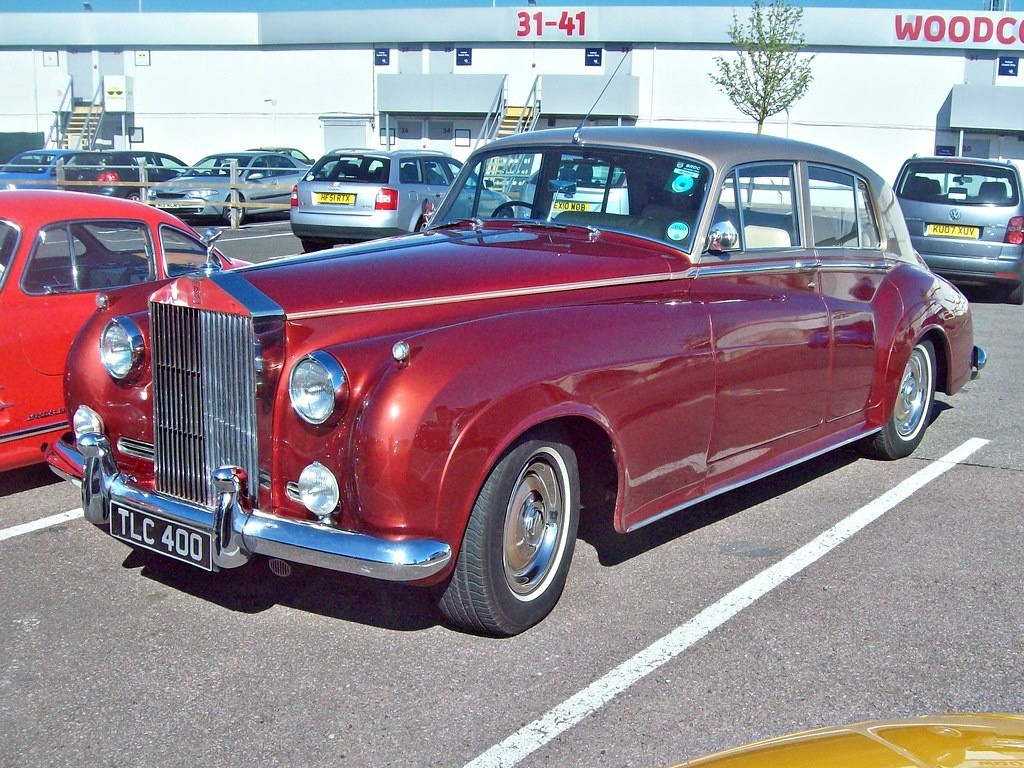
519 153 633 226
288 147 514 254
60 151 201 205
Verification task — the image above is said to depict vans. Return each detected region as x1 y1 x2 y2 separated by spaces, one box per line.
891 150 1024 301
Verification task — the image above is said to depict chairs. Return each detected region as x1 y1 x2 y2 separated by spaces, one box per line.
974 182 1010 202
813 215 870 247
733 225 791 249
921 180 944 201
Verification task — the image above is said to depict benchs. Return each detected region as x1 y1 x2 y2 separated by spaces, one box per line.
551 210 664 240
727 209 796 246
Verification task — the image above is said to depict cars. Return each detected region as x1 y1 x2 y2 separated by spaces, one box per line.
147 151 309 226
0 188 259 474
46 130 989 636
0 150 82 189
243 146 327 178
